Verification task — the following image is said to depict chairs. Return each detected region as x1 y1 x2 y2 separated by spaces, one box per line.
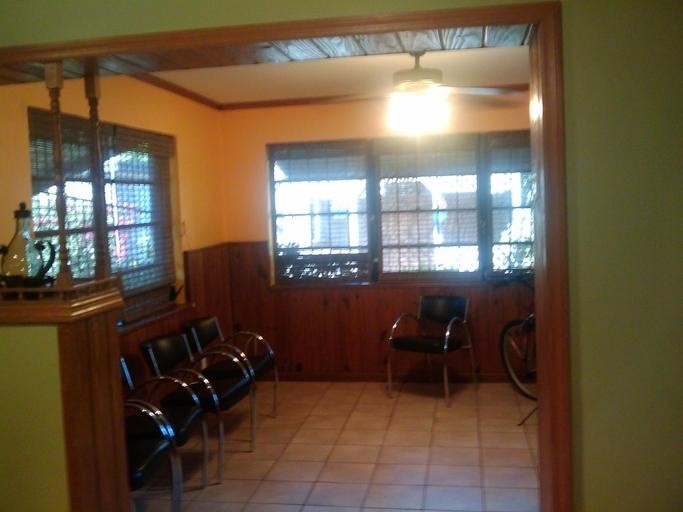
385 295 478 407
120 315 279 512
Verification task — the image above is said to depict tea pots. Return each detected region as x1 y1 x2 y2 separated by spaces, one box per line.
0 202 55 278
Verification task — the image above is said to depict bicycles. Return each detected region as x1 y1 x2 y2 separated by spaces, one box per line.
494 269 538 425
